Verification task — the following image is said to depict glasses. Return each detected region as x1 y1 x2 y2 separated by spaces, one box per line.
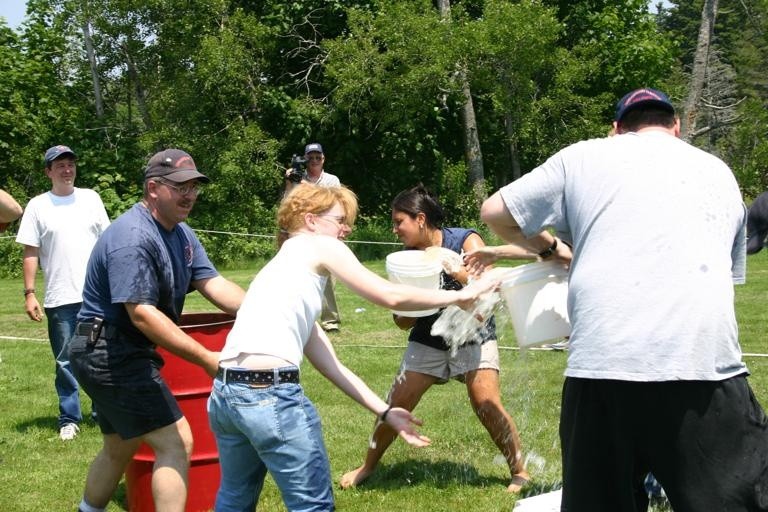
312 213 350 225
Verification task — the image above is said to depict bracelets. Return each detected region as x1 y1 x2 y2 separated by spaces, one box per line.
381 404 394 420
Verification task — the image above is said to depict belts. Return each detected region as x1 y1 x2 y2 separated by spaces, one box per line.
215 366 301 384
77 321 156 350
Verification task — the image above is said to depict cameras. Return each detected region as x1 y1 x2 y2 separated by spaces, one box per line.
288 154 308 184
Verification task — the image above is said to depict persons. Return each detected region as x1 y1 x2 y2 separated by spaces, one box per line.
205 185 497 512
734 188 768 255
280 144 343 330
16 145 110 440
340 187 532 500
68 149 246 512
461 203 572 278
0 187 23 231
480 87 767 512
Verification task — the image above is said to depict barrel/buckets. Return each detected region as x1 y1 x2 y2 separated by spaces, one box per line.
125 313 236 512
500 259 571 348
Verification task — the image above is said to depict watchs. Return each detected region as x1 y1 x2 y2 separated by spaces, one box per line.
24 289 36 295
538 236 562 259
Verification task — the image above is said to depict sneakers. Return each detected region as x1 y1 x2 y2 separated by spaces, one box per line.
59 420 78 442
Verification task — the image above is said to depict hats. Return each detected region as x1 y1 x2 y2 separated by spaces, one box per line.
616 88 669 119
145 149 210 183
44 145 75 162
305 143 322 155
